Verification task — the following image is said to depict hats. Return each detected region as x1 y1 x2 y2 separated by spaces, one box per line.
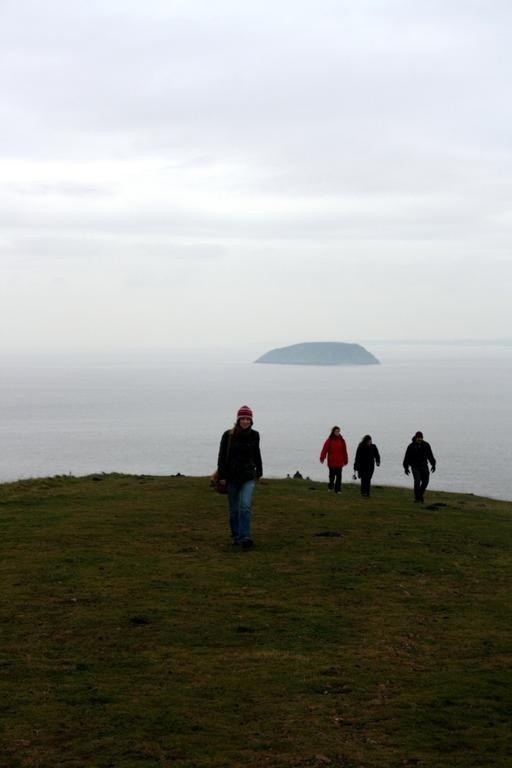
237 405 253 420
416 431 423 438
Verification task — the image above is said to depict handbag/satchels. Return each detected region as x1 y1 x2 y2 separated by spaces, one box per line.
209 472 227 494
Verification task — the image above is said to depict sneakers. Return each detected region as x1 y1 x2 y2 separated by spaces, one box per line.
231 536 254 546
328 489 369 499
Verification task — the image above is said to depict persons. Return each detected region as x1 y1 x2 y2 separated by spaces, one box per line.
401 430 437 504
320 424 348 495
216 404 265 546
352 433 381 498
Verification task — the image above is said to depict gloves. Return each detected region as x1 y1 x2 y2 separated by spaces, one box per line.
405 469 410 476
431 466 435 473
